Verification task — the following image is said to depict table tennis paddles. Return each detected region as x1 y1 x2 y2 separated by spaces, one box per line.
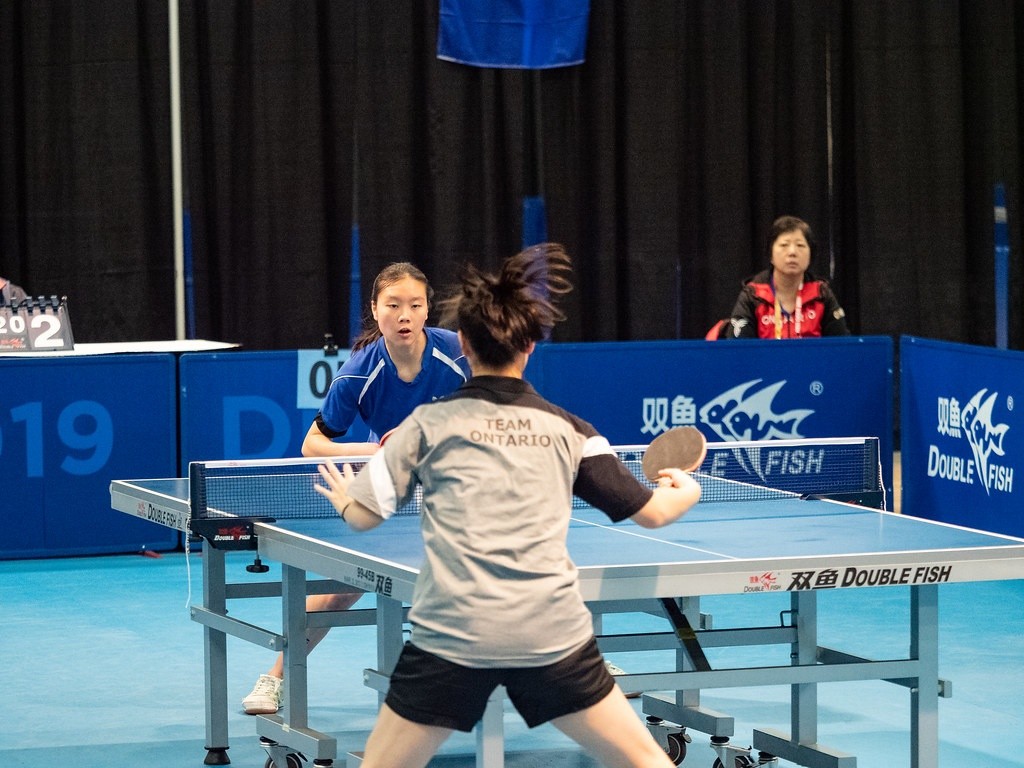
642 425 707 489
380 426 401 447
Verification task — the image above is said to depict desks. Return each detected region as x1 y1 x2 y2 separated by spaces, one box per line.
109 461 1024 768
0 340 243 357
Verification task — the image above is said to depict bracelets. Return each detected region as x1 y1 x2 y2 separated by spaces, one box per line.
340 501 353 522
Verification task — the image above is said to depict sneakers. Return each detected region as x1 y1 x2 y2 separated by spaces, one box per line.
242 674 284 714
605 661 642 697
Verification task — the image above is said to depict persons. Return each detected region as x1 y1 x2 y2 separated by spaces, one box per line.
312 240 708 767
724 215 851 341
239 260 473 716
0 278 27 308
